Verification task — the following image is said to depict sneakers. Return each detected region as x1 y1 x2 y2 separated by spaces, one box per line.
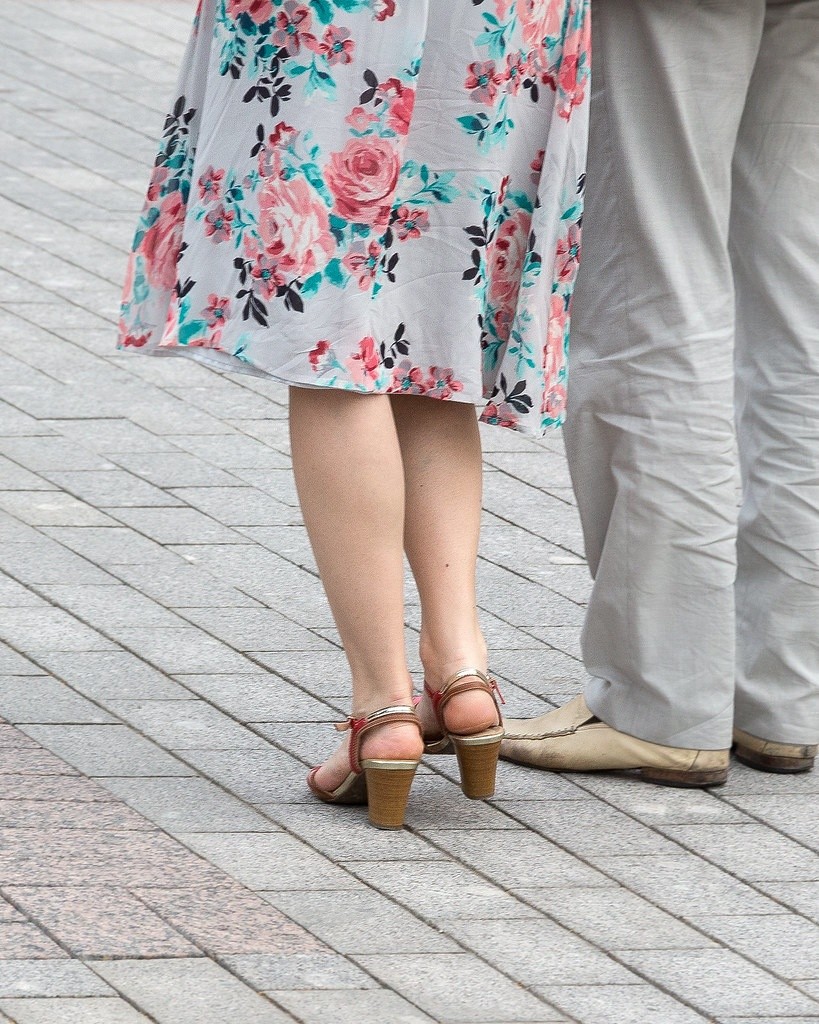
733 728 817 773
501 692 728 786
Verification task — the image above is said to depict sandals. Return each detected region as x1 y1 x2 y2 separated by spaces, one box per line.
413 667 502 801
307 703 427 830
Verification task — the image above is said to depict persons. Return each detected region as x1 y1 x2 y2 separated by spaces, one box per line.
111 0 605 839
488 0 819 794
